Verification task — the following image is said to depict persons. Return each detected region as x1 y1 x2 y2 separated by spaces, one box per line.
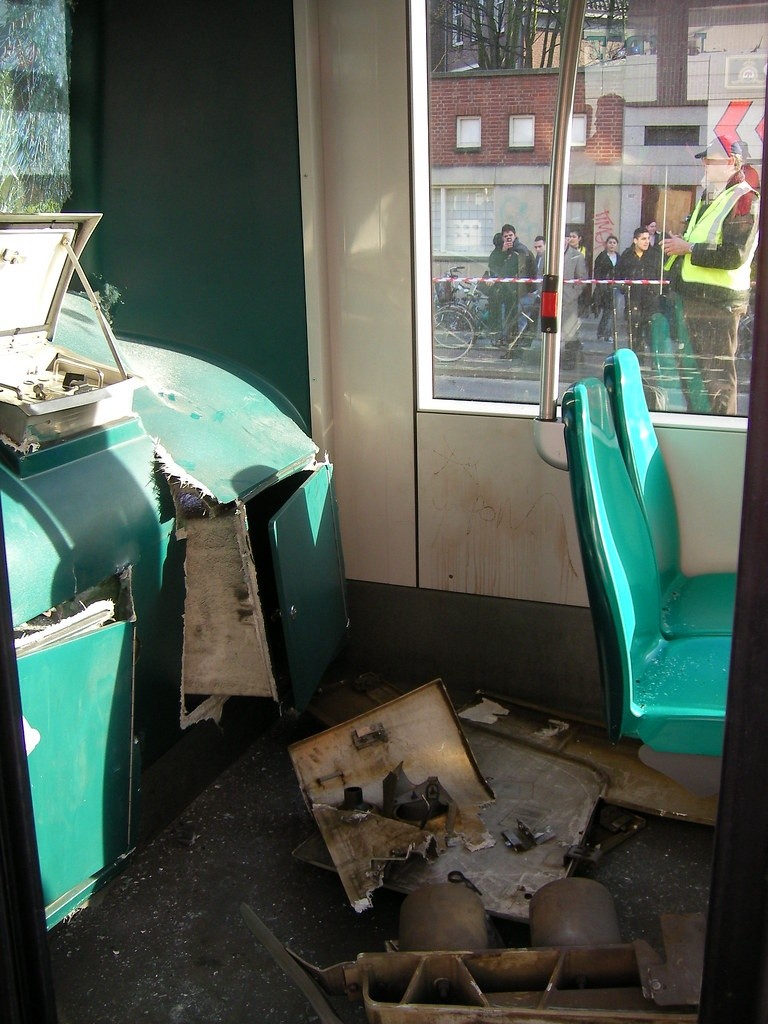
658 135 762 415
487 218 756 361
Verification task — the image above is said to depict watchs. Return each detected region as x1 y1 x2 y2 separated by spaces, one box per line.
689 244 695 252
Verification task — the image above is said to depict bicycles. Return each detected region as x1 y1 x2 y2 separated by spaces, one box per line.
431 265 542 365
736 314 754 394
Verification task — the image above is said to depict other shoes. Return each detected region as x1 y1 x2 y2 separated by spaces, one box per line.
598 334 614 344
562 349 577 371
499 351 521 359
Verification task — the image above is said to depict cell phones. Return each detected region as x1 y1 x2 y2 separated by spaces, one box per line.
507 238 511 242
660 232 671 241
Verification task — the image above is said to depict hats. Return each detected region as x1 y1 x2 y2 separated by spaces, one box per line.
694 137 742 159
730 140 754 158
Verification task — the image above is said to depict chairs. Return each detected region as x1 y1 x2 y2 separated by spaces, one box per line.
562 349 738 755
